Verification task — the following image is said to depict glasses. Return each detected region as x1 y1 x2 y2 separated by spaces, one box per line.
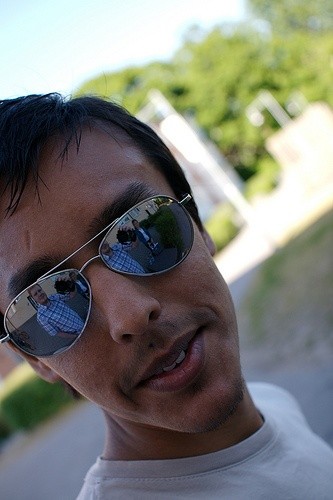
0 193 194 359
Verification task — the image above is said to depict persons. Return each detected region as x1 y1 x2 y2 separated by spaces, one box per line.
131 218 159 255
0 93 333 500
27 284 85 338
99 239 145 278
